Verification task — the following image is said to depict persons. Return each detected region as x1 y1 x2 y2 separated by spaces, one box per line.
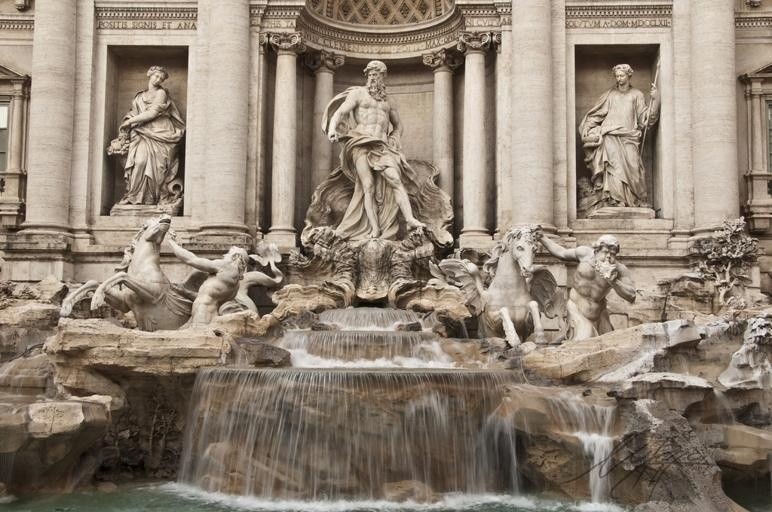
164 234 252 334
535 229 636 344
328 58 427 242
576 62 660 207
117 64 185 205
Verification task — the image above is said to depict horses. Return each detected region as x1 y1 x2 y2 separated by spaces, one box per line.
60 214 193 332
477 224 550 350
